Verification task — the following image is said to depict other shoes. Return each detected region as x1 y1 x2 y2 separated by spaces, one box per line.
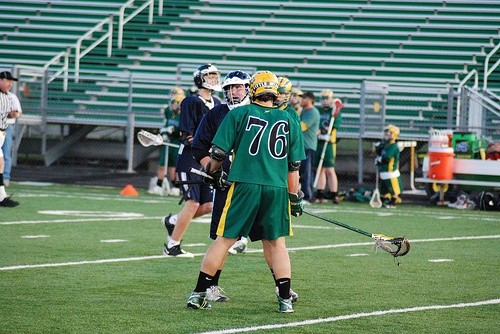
446 192 476 210
333 195 339 203
313 197 326 204
0 193 20 207
146 185 163 195
381 196 397 209
168 187 180 196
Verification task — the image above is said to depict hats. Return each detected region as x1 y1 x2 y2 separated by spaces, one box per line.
0 70 19 82
299 92 314 99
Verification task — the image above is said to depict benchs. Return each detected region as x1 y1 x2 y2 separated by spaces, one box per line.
0 0 500 143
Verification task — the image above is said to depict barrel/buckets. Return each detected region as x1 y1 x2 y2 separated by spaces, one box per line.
428 147 454 181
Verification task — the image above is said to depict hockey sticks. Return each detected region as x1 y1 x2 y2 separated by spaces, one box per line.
370 156 384 207
187 166 410 258
312 97 344 188
137 128 181 151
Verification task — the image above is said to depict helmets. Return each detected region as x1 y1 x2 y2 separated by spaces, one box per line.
291 88 302 105
192 63 222 92
384 124 400 142
319 87 333 104
277 76 293 108
170 94 186 101
249 71 279 97
171 87 184 94
222 70 251 110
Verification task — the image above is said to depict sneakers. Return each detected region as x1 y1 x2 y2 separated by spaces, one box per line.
205 285 229 302
277 298 295 313
274 287 299 299
161 212 175 242
228 237 248 255
184 291 211 308
162 244 196 258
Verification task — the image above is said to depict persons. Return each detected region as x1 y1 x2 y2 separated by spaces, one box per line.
148 64 341 302
368 124 403 209
186 69 307 313
0 70 22 208
